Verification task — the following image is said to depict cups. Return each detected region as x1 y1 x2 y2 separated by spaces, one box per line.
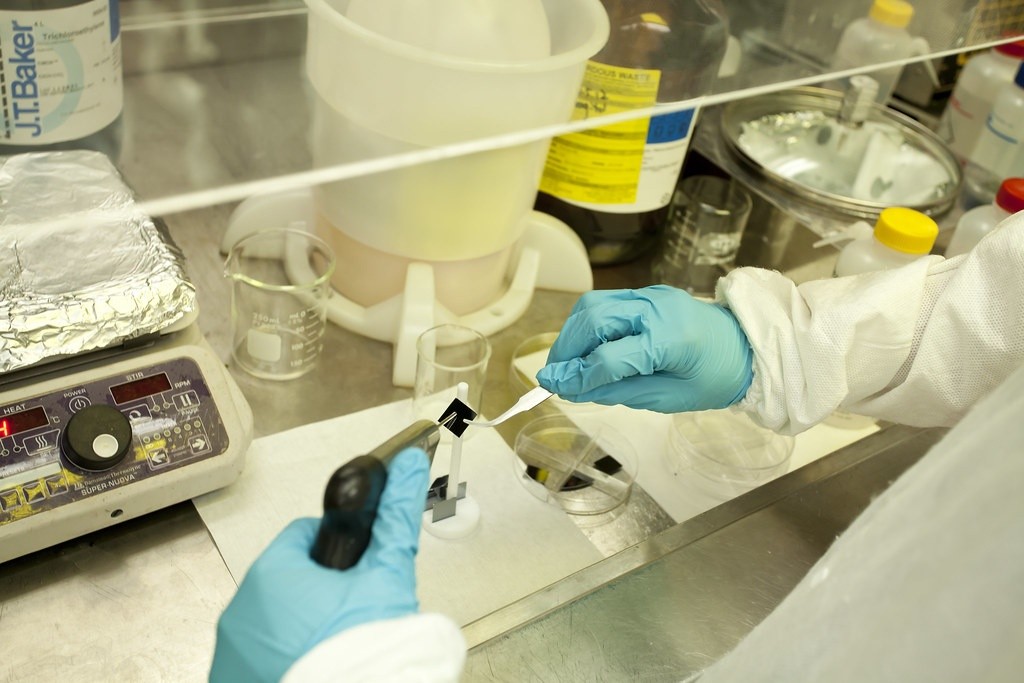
650 176 751 297
414 326 490 441
221 229 335 381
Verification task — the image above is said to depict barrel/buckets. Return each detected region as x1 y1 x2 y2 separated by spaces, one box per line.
302 0 610 316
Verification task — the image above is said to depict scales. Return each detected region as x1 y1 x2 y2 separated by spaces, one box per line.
1 146 256 566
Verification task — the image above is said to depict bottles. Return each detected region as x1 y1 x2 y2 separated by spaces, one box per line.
0 0 124 165
533 0 728 270
820 0 914 116
936 29 1024 164
964 61 1024 194
945 177 1024 257
832 207 938 278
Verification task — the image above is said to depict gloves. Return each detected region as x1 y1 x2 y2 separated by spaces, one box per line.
535 284 755 414
206 444 433 683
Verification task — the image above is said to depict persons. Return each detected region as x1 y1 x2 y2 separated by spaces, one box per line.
209 212 1016 683
